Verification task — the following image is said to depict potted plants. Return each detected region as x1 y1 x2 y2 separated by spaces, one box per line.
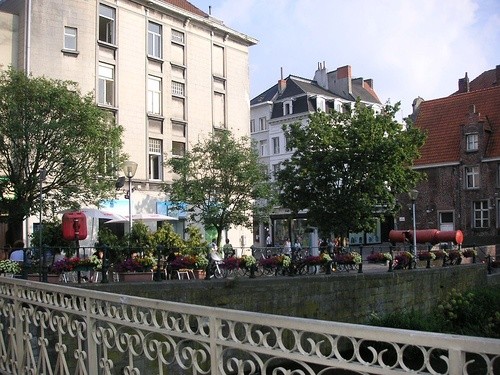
0 258 23 278
195 256 210 279
170 254 194 270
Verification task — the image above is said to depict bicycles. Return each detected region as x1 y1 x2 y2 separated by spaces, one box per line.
230 248 360 277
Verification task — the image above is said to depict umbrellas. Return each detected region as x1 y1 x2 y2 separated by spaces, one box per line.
104 213 178 224
57 207 128 221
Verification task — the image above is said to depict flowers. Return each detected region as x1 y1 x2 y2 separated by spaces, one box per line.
461 248 477 258
305 255 322 266
429 250 448 260
116 256 156 272
415 250 430 260
49 254 103 273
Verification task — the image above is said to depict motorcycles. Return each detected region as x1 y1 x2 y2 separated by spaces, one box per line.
207 250 228 280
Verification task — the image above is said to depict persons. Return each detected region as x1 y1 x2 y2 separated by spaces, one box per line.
295 240 302 249
223 239 232 256
284 238 291 256
319 235 349 255
211 238 217 251
265 234 271 246
10 240 25 261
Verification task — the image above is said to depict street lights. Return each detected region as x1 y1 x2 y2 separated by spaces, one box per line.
121 161 137 248
34 167 48 282
408 189 419 265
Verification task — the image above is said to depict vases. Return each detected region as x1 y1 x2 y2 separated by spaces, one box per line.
179 269 194 280
462 256 473 264
119 273 154 282
430 258 444 267
415 260 428 270
306 265 320 275
61 270 103 284
